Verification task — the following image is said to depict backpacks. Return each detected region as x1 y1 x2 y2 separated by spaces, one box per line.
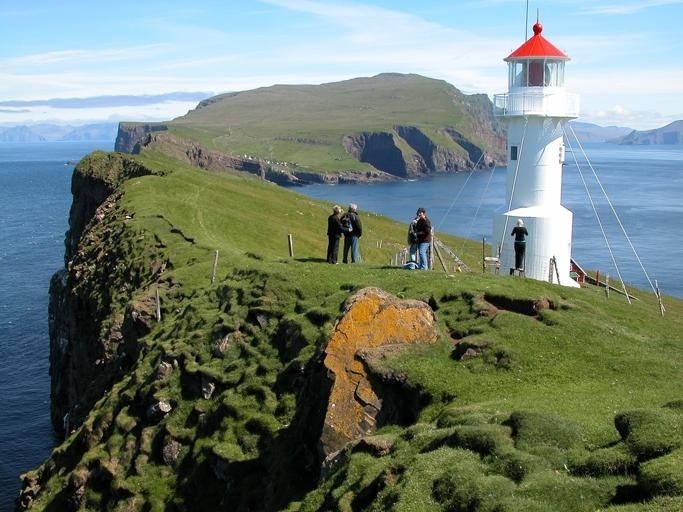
342 215 355 232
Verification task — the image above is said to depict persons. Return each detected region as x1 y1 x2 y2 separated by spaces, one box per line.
340 204 362 263
417 208 430 270
325 203 351 264
511 219 527 270
408 212 418 262
